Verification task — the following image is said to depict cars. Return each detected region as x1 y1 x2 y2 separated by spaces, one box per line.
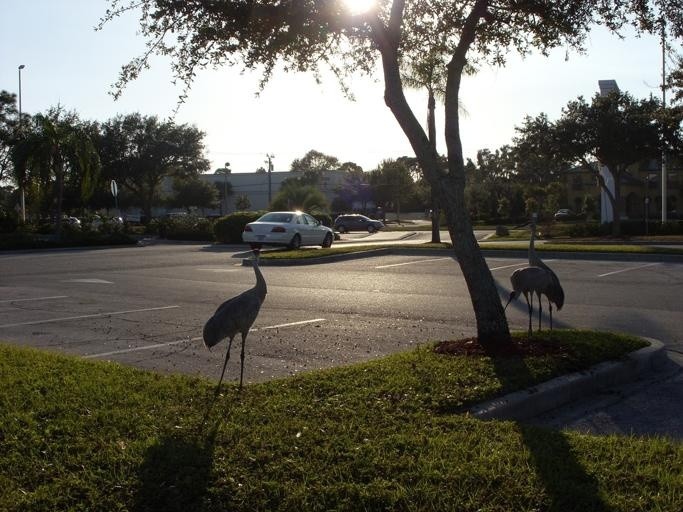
165 211 222 228
555 209 575 220
241 211 335 251
64 215 124 227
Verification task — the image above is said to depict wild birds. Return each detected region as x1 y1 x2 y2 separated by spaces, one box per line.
503 266 560 338
527 222 565 334
203 242 268 396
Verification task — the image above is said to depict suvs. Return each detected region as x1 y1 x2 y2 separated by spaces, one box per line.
334 213 385 234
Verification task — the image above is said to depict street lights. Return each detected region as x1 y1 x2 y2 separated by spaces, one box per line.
15 62 29 225
225 162 233 214
264 153 275 207
